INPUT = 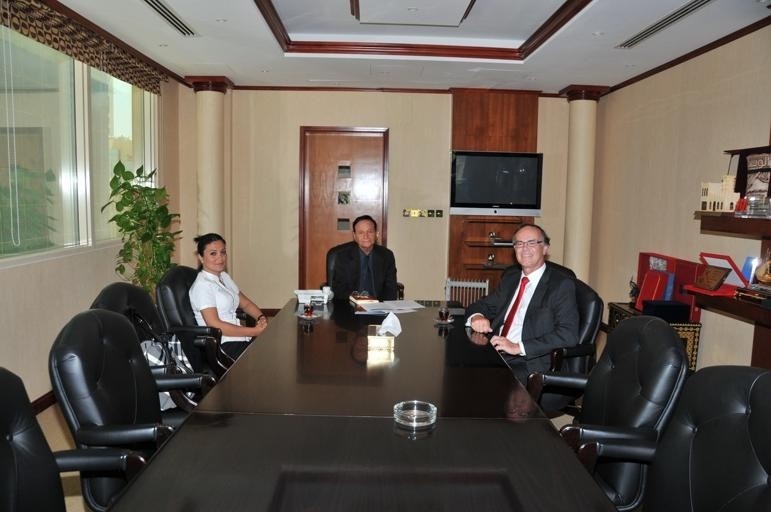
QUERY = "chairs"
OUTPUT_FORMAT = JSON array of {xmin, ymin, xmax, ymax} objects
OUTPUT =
[
  {"xmin": 324, "ymin": 239, "xmax": 405, "ymax": 301},
  {"xmin": 49, "ymin": 308, "xmax": 191, "ymax": 512},
  {"xmin": 87, "ymin": 280, "xmax": 219, "ymax": 421},
  {"xmin": 526, "ymin": 276, "xmax": 603, "ymax": 414},
  {"xmin": 0, "ymin": 367, "xmax": 146, "ymax": 512},
  {"xmin": 577, "ymin": 364, "xmax": 770, "ymax": 511},
  {"xmin": 529, "ymin": 315, "xmax": 689, "ymax": 511},
  {"xmin": 154, "ymin": 265, "xmax": 252, "ymax": 369}
]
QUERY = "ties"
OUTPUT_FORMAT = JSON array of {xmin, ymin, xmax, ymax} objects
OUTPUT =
[
  {"xmin": 363, "ymin": 255, "xmax": 374, "ymax": 296},
  {"xmin": 501, "ymin": 277, "xmax": 530, "ymax": 338}
]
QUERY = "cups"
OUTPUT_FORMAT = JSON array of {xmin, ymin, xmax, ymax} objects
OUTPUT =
[
  {"xmin": 304, "ymin": 303, "xmax": 314, "ymax": 316},
  {"xmin": 438, "ymin": 307, "xmax": 450, "ymax": 322}
]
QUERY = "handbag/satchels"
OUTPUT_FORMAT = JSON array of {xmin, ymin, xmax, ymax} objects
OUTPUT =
[{"xmin": 125, "ymin": 306, "xmax": 196, "ymax": 411}]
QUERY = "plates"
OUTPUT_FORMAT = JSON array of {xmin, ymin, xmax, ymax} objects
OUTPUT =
[
  {"xmin": 298, "ymin": 314, "xmax": 318, "ymax": 320},
  {"xmin": 434, "ymin": 319, "xmax": 454, "ymax": 324}
]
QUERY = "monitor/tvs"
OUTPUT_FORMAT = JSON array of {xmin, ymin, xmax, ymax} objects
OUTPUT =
[{"xmin": 448, "ymin": 150, "xmax": 544, "ymax": 217}]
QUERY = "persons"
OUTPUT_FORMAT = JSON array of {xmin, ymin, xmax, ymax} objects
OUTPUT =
[
  {"xmin": 464, "ymin": 224, "xmax": 579, "ymax": 387},
  {"xmin": 331, "ymin": 215, "xmax": 397, "ymax": 302},
  {"xmin": 187, "ymin": 234, "xmax": 268, "ymax": 361}
]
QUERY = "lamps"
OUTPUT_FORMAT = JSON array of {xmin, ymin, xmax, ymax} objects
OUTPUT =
[{"xmin": 359, "ymin": 0, "xmax": 472, "ymax": 27}]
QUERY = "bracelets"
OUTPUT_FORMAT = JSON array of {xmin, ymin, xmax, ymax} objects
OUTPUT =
[{"xmin": 258, "ymin": 314, "xmax": 267, "ymax": 320}]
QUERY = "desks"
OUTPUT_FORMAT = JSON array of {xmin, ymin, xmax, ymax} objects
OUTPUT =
[
  {"xmin": 97, "ymin": 416, "xmax": 617, "ymax": 512},
  {"xmin": 190, "ymin": 298, "xmax": 546, "ymax": 419}
]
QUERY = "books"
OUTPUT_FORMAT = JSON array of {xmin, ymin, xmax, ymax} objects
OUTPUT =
[{"xmin": 348, "ymin": 294, "xmax": 379, "ymax": 304}]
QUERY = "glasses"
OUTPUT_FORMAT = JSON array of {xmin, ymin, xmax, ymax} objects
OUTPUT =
[{"xmin": 513, "ymin": 239, "xmax": 546, "ymax": 247}]
